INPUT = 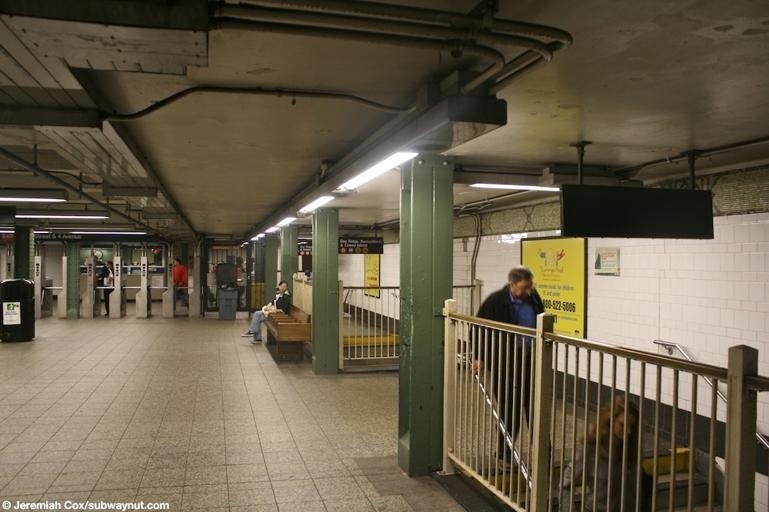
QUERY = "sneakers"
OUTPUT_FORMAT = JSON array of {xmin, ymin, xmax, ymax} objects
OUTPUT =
[
  {"xmin": 497, "ymin": 458, "xmax": 514, "ymax": 472},
  {"xmin": 241, "ymin": 329, "xmax": 263, "ymax": 344}
]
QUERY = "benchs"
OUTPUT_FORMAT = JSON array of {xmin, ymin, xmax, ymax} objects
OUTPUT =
[{"xmin": 262, "ymin": 304, "xmax": 313, "ymax": 362}]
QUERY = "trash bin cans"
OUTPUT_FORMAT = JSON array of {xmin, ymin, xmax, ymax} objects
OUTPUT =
[
  {"xmin": 216, "ymin": 263, "xmax": 239, "ymax": 321},
  {"xmin": 1, "ymin": 279, "xmax": 35, "ymax": 342}
]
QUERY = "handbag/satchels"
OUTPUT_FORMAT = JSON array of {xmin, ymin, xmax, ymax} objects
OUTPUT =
[{"xmin": 102, "ymin": 270, "xmax": 114, "ymax": 288}]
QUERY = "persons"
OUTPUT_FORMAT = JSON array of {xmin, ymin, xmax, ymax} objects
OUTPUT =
[
  {"xmin": 241, "ymin": 280, "xmax": 291, "ymax": 344},
  {"xmin": 559, "ymin": 395, "xmax": 639, "ymax": 512},
  {"xmin": 235, "ymin": 256, "xmax": 247, "ymax": 305},
  {"xmin": 469, "ymin": 265, "xmax": 551, "ymax": 476},
  {"xmin": 173, "ymin": 258, "xmax": 189, "ymax": 305},
  {"xmin": 103, "ymin": 261, "xmax": 114, "ymax": 318}
]
